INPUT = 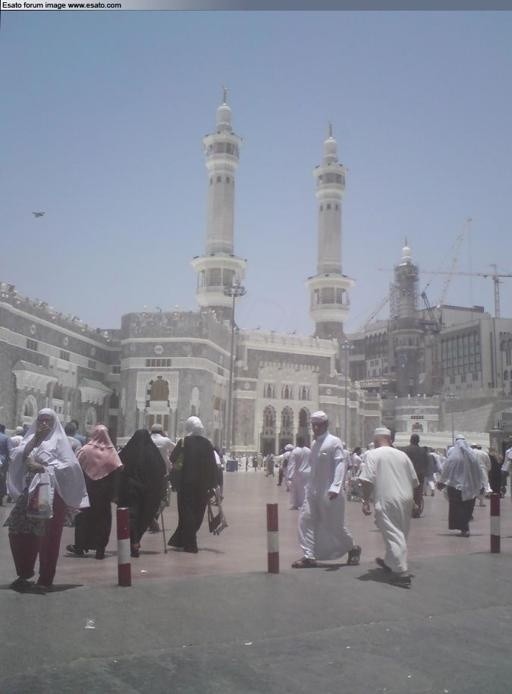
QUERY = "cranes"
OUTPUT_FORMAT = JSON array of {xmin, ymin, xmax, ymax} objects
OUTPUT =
[{"xmin": 360, "ymin": 216, "xmax": 512, "ymax": 330}]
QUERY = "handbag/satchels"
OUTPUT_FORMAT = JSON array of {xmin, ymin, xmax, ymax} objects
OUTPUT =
[
  {"xmin": 169, "ymin": 447, "xmax": 184, "ymax": 492},
  {"xmin": 26, "ymin": 466, "xmax": 55, "ymax": 519}
]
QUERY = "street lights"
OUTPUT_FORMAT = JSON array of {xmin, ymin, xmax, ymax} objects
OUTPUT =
[
  {"xmin": 446, "ymin": 390, "xmax": 457, "ymax": 447},
  {"xmin": 341, "ymin": 338, "xmax": 355, "ymax": 450},
  {"xmin": 223, "ymin": 278, "xmax": 246, "ymax": 456}
]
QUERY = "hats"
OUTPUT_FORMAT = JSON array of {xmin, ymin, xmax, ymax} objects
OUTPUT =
[
  {"xmin": 310, "ymin": 410, "xmax": 328, "ymax": 423},
  {"xmin": 374, "ymin": 428, "xmax": 391, "ymax": 436}
]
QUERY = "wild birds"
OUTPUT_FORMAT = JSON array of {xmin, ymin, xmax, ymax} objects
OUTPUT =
[{"xmin": 32, "ymin": 212, "xmax": 45, "ymax": 217}]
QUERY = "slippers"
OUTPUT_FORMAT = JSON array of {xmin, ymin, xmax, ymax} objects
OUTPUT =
[{"xmin": 67, "ymin": 544, "xmax": 84, "ymax": 555}]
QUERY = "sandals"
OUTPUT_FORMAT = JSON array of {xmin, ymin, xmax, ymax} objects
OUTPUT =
[
  {"xmin": 292, "ymin": 557, "xmax": 318, "ymax": 568},
  {"xmin": 347, "ymin": 546, "xmax": 361, "ymax": 565}
]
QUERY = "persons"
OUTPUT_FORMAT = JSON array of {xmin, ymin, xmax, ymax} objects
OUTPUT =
[{"xmin": 1, "ymin": 408, "xmax": 512, "ymax": 593}]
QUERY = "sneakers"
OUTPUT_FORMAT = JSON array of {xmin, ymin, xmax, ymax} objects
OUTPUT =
[
  {"xmin": 376, "ymin": 558, "xmax": 411, "ymax": 586},
  {"xmin": 11, "ymin": 576, "xmax": 50, "ymax": 594}
]
